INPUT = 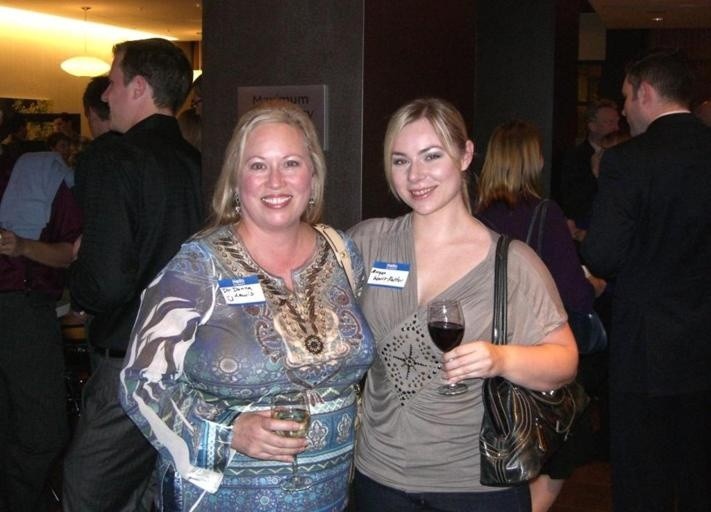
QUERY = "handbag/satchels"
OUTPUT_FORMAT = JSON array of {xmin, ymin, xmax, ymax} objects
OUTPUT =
[
  {"xmin": 523, "ymin": 197, "xmax": 608, "ymax": 353},
  {"xmin": 475, "ymin": 229, "xmax": 590, "ymax": 490}
]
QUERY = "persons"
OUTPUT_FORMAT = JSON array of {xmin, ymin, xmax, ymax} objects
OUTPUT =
[
  {"xmin": 117, "ymin": 100, "xmax": 377, "ymax": 512},
  {"xmin": 343, "ymin": 97, "xmax": 582, "ymax": 512},
  {"xmin": 474, "ymin": 118, "xmax": 607, "ymax": 512},
  {"xmin": 60, "ymin": 35, "xmax": 201, "ymax": 511},
  {"xmin": 551, "ymin": 96, "xmax": 710, "ymax": 242},
  {"xmin": 579, "ymin": 45, "xmax": 711, "ymax": 512}
]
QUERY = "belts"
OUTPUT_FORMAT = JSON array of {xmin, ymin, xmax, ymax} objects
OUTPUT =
[{"xmin": 92, "ymin": 346, "xmax": 126, "ymax": 360}]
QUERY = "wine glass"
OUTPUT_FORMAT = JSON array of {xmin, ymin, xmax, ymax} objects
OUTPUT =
[
  {"xmin": 425, "ymin": 297, "xmax": 469, "ymax": 398},
  {"xmin": 268, "ymin": 389, "xmax": 318, "ymax": 495}
]
